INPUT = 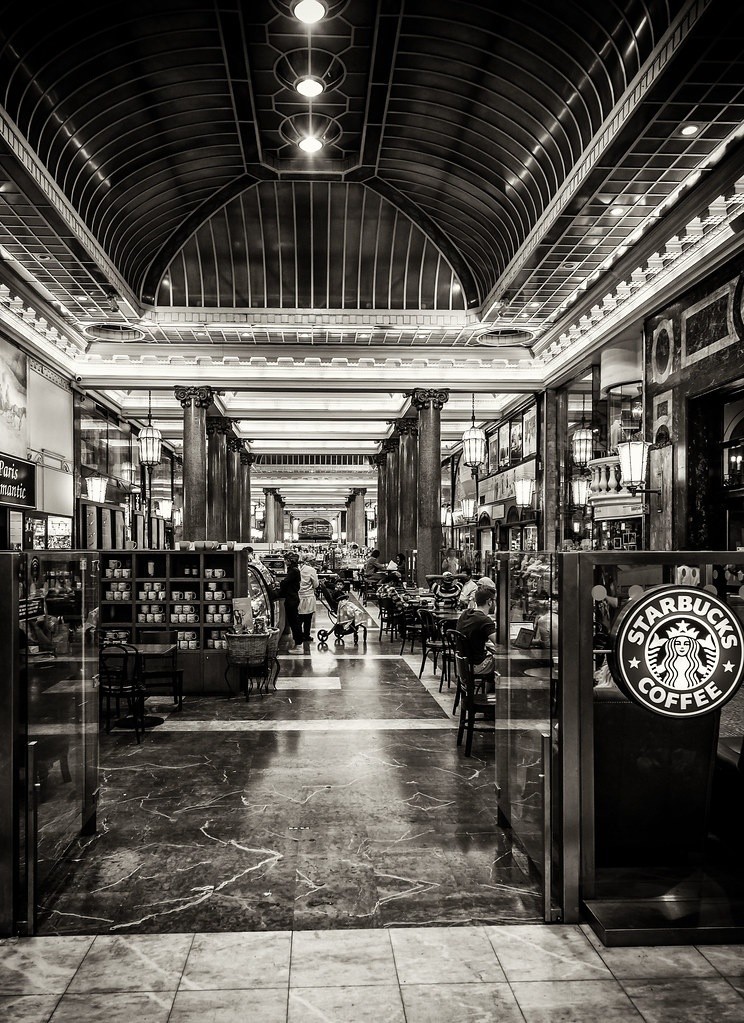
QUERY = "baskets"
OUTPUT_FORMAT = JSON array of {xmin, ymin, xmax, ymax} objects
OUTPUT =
[
  {"xmin": 266, "ymin": 627, "xmax": 280, "ymax": 659},
  {"xmin": 227, "ymin": 632, "xmax": 268, "ymax": 665}
]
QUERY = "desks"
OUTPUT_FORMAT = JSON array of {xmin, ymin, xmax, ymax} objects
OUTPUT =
[
  {"xmin": 436, "ymin": 609, "xmax": 463, "ymax": 624},
  {"xmin": 101, "ymin": 642, "xmax": 177, "ymax": 728},
  {"xmin": 484, "ymin": 643, "xmax": 550, "ymax": 678}
]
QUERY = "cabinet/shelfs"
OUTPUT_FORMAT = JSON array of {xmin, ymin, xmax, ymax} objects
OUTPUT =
[{"xmin": 70, "ymin": 548, "xmax": 253, "ymax": 691}]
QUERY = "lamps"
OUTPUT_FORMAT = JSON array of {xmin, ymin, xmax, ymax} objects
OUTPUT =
[
  {"xmin": 363, "ymin": 394, "xmax": 642, "ymax": 549},
  {"xmin": 86, "ymin": 386, "xmax": 300, "ymax": 541}
]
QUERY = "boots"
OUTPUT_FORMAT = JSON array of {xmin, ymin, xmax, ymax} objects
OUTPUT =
[{"xmin": 288, "ymin": 643, "xmax": 304, "ymax": 655}]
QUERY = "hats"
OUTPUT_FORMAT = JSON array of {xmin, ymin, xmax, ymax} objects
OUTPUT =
[
  {"xmin": 303, "ymin": 553, "xmax": 316, "ymax": 562},
  {"xmin": 476, "ymin": 576, "xmax": 496, "ymax": 588},
  {"xmin": 475, "ymin": 584, "xmax": 495, "ymax": 600}
]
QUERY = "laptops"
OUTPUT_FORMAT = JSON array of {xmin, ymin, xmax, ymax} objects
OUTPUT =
[{"xmin": 509, "ymin": 621, "xmax": 534, "ymax": 650}]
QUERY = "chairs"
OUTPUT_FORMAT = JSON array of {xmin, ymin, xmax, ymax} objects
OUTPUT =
[
  {"xmin": 96, "ymin": 629, "xmax": 183, "ymax": 740},
  {"xmin": 356, "ymin": 566, "xmax": 496, "ymax": 757}
]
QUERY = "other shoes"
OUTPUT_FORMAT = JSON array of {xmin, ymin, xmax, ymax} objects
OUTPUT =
[{"xmin": 304, "ymin": 635, "xmax": 313, "ymax": 641}]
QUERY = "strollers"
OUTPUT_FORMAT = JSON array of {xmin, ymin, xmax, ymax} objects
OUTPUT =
[{"xmin": 314, "ymin": 578, "xmax": 368, "ymax": 646}]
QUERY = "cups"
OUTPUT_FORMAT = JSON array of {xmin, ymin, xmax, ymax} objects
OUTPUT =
[
  {"xmin": 204, "ymin": 583, "xmax": 233, "ymax": 600},
  {"xmin": 192, "ymin": 569, "xmax": 198, "ymax": 575},
  {"xmin": 105, "ymin": 560, "xmax": 131, "ymax": 578},
  {"xmin": 125, "ymin": 540, "xmax": 137, "ymax": 550},
  {"xmin": 205, "ymin": 568, "xmax": 214, "ymax": 578},
  {"xmin": 137, "ymin": 605, "xmax": 165, "ymax": 622},
  {"xmin": 139, "ymin": 582, "xmax": 166, "ymax": 601},
  {"xmin": 227, "ymin": 541, "xmax": 236, "ymax": 551},
  {"xmin": 207, "ymin": 630, "xmax": 229, "ymax": 649},
  {"xmin": 185, "ymin": 592, "xmax": 196, "ymax": 600},
  {"xmin": 170, "ymin": 605, "xmax": 199, "ymax": 623},
  {"xmin": 184, "ymin": 568, "xmax": 190, "ymax": 574},
  {"xmin": 215, "ymin": 569, "xmax": 226, "ymax": 578},
  {"xmin": 102, "ymin": 629, "xmax": 130, "ymax": 646},
  {"xmin": 179, "ymin": 540, "xmax": 193, "ymax": 550},
  {"xmin": 176, "ymin": 631, "xmax": 200, "ymax": 650},
  {"xmin": 193, "ymin": 541, "xmax": 219, "ymax": 551},
  {"xmin": 206, "ymin": 605, "xmax": 231, "ymax": 622},
  {"xmin": 172, "ymin": 591, "xmax": 184, "ymax": 600},
  {"xmin": 582, "ymin": 538, "xmax": 592, "ymax": 551},
  {"xmin": 106, "ymin": 583, "xmax": 132, "ymax": 600}
]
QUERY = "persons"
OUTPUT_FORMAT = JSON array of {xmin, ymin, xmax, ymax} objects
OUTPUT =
[
  {"xmin": 455, "ymin": 584, "xmax": 496, "ymax": 674},
  {"xmin": 531, "ymin": 603, "xmax": 559, "ymax": 648},
  {"xmin": 442, "ymin": 548, "xmax": 461, "ymax": 574},
  {"xmin": 246, "ymin": 547, "xmax": 259, "ymax": 559},
  {"xmin": 425, "ymin": 567, "xmax": 477, "ymax": 609},
  {"xmin": 363, "ymin": 550, "xmax": 387, "ymax": 585},
  {"xmin": 273, "ymin": 551, "xmax": 303, "ymax": 645},
  {"xmin": 395, "ymin": 553, "xmax": 405, "ymax": 575},
  {"xmin": 332, "ymin": 579, "xmax": 345, "ymax": 600},
  {"xmin": 299, "ymin": 553, "xmax": 319, "ymax": 642}
]
